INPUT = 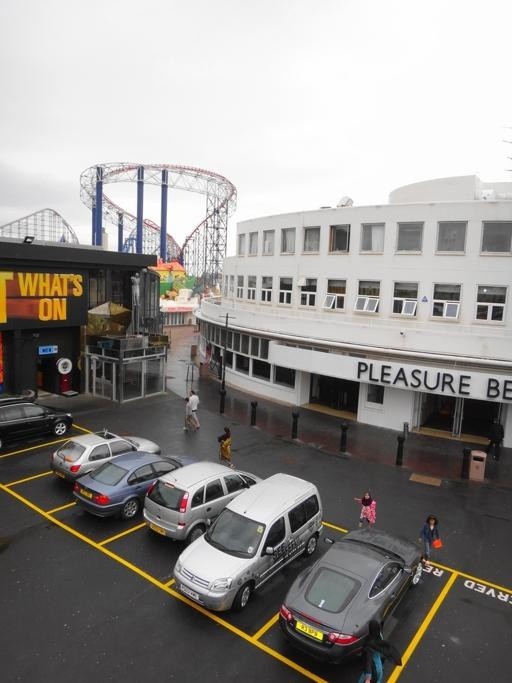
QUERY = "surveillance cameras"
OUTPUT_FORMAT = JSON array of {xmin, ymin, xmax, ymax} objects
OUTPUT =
[{"xmin": 399, "ymin": 331, "xmax": 405, "ymax": 336}]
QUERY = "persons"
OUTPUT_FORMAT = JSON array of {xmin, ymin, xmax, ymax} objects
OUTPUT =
[
  {"xmin": 353, "ymin": 490, "xmax": 376, "ymax": 528},
  {"xmin": 218, "ymin": 427, "xmax": 234, "ymax": 468},
  {"xmin": 485, "ymin": 417, "xmax": 504, "ymax": 461},
  {"xmin": 189, "ymin": 391, "xmax": 200, "ymax": 430},
  {"xmin": 130, "ymin": 273, "xmax": 141, "ymax": 306},
  {"xmin": 357, "ymin": 620, "xmax": 402, "ymax": 683},
  {"xmin": 183, "ymin": 397, "xmax": 196, "ymax": 433},
  {"xmin": 418, "ymin": 514, "xmax": 440, "ymax": 561}
]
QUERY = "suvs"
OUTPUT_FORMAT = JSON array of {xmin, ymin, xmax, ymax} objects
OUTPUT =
[
  {"xmin": 52, "ymin": 428, "xmax": 161, "ymax": 485},
  {"xmin": 0, "ymin": 397, "xmax": 74, "ymax": 446},
  {"xmin": 143, "ymin": 460, "xmax": 263, "ymax": 544},
  {"xmin": 171, "ymin": 472, "xmax": 324, "ymax": 613},
  {"xmin": 280, "ymin": 526, "xmax": 428, "ymax": 664}
]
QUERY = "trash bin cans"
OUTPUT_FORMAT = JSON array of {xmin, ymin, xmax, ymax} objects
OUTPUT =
[
  {"xmin": 469, "ymin": 450, "xmax": 488, "ymax": 481},
  {"xmin": 200, "ymin": 360, "xmax": 209, "ymax": 376}
]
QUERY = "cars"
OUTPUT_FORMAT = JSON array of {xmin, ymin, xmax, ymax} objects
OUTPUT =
[{"xmin": 72, "ymin": 449, "xmax": 200, "ymax": 520}]
